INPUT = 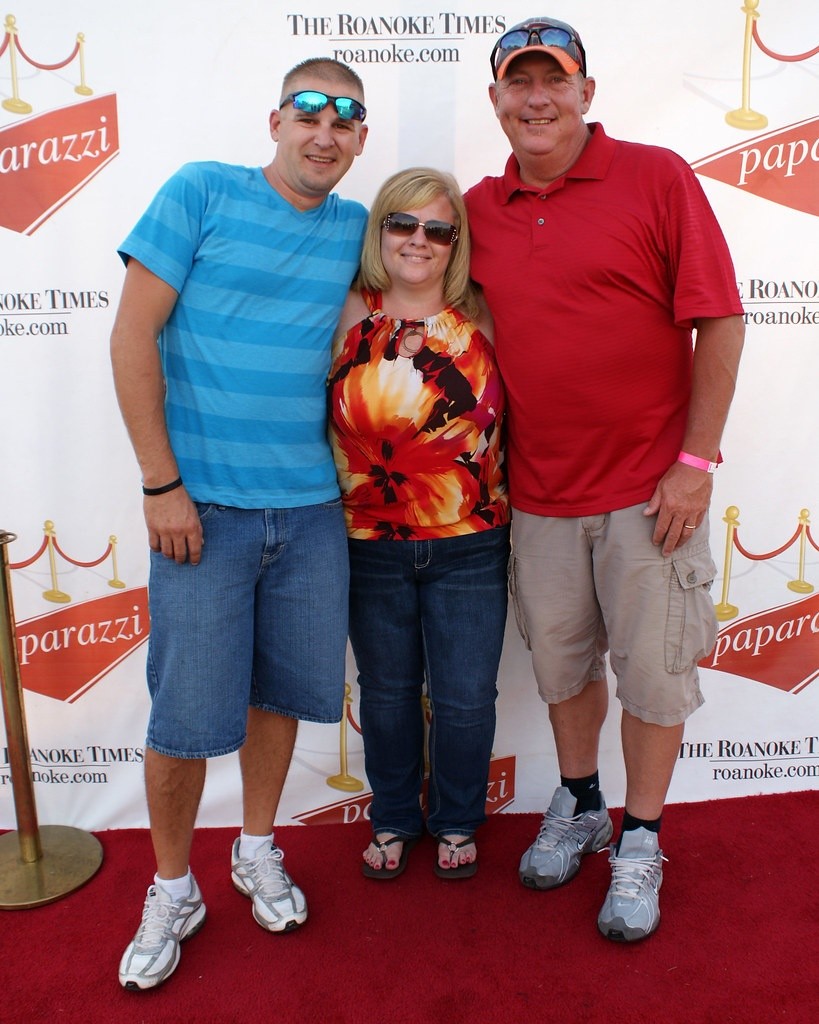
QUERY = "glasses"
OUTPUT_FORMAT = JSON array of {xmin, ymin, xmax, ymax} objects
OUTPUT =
[
  {"xmin": 490, "ymin": 26, "xmax": 587, "ymax": 82},
  {"xmin": 279, "ymin": 89, "xmax": 367, "ymax": 123},
  {"xmin": 381, "ymin": 212, "xmax": 459, "ymax": 246}
]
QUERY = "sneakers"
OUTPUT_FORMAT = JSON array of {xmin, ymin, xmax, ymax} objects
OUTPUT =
[
  {"xmin": 598, "ymin": 826, "xmax": 669, "ymax": 943},
  {"xmin": 231, "ymin": 837, "xmax": 308, "ymax": 935},
  {"xmin": 118, "ymin": 873, "xmax": 206, "ymax": 991},
  {"xmin": 518, "ymin": 786, "xmax": 613, "ymax": 889}
]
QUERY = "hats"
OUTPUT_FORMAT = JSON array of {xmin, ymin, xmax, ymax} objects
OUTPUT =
[{"xmin": 495, "ymin": 16, "xmax": 585, "ymax": 81}]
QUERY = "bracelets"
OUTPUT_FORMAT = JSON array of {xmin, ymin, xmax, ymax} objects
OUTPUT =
[
  {"xmin": 677, "ymin": 451, "xmax": 721, "ymax": 475},
  {"xmin": 140, "ymin": 475, "xmax": 184, "ymax": 495}
]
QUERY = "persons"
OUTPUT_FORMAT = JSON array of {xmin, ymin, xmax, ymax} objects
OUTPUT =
[
  {"xmin": 323, "ymin": 165, "xmax": 515, "ymax": 881},
  {"xmin": 104, "ymin": 59, "xmax": 371, "ymax": 990},
  {"xmin": 457, "ymin": 13, "xmax": 745, "ymax": 946}
]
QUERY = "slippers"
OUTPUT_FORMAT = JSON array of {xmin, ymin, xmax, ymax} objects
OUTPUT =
[
  {"xmin": 361, "ymin": 827, "xmax": 409, "ymax": 878},
  {"xmin": 434, "ymin": 833, "xmax": 478, "ymax": 878}
]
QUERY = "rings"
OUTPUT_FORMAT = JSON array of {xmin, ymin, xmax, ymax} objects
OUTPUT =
[{"xmin": 684, "ymin": 524, "xmax": 697, "ymax": 530}]
